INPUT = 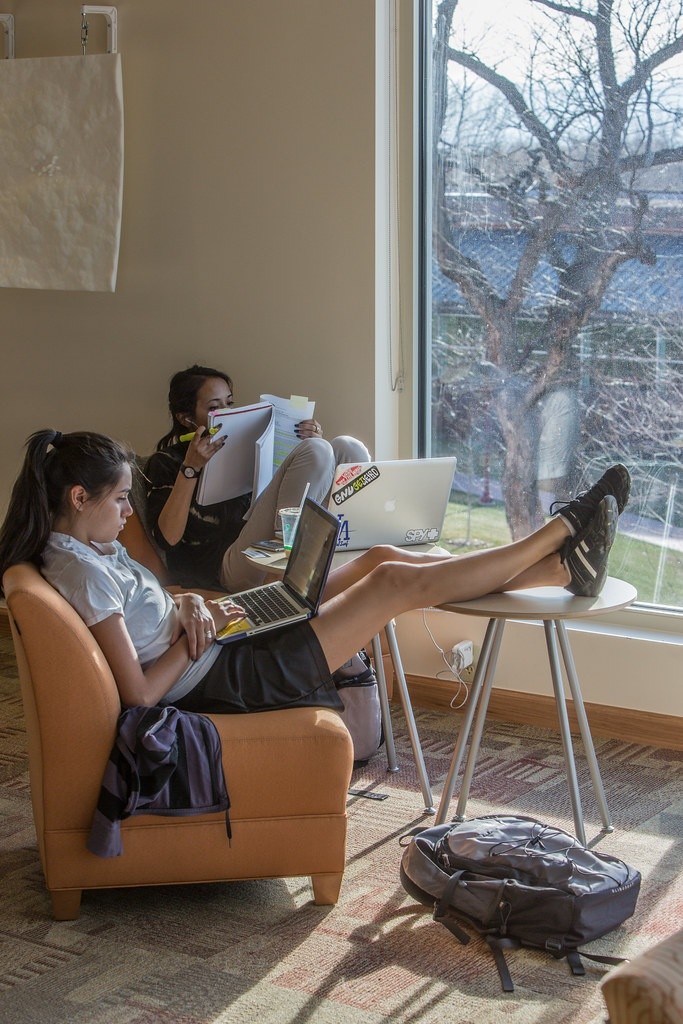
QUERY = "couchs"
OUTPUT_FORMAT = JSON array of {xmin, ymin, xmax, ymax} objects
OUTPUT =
[
  {"xmin": 2, "ymin": 560, "xmax": 354, "ymax": 921},
  {"xmin": 118, "ymin": 454, "xmax": 394, "ymax": 700}
]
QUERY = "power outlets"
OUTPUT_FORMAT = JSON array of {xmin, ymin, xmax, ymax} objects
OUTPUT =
[{"xmin": 459, "ymin": 645, "xmax": 479, "ymax": 684}]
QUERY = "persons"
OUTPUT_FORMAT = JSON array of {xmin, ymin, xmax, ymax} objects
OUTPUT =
[
  {"xmin": 1, "ymin": 429, "xmax": 632, "ymax": 714},
  {"xmin": 142, "ymin": 365, "xmax": 371, "ymax": 594}
]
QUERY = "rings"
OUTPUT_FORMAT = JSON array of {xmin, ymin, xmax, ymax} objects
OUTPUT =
[{"xmin": 205, "ymin": 630, "xmax": 213, "ymax": 638}]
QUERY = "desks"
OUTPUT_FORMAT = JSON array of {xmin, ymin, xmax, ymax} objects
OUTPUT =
[
  {"xmin": 434, "ymin": 574, "xmax": 638, "ymax": 849},
  {"xmin": 247, "ymin": 544, "xmax": 451, "ymax": 814}
]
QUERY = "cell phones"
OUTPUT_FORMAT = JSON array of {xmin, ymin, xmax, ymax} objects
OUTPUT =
[{"xmin": 250, "ymin": 541, "xmax": 284, "ymax": 553}]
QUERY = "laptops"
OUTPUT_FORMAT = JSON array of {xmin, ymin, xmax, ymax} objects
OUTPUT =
[
  {"xmin": 274, "ymin": 457, "xmax": 458, "ymax": 552},
  {"xmin": 205, "ymin": 498, "xmax": 343, "ymax": 646}
]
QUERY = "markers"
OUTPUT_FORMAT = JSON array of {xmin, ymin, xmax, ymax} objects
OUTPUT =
[{"xmin": 180, "ymin": 428, "xmax": 219, "ymax": 442}]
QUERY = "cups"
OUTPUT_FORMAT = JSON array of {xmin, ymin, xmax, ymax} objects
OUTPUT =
[{"xmin": 278, "ymin": 507, "xmax": 300, "ymax": 558}]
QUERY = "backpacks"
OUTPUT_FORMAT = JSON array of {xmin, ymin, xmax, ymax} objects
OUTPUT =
[
  {"xmin": 332, "ymin": 649, "xmax": 384, "ymax": 771},
  {"xmin": 397, "ymin": 814, "xmax": 641, "ymax": 992}
]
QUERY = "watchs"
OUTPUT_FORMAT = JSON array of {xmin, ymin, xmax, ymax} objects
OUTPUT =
[{"xmin": 180, "ymin": 464, "xmax": 203, "ymax": 479}]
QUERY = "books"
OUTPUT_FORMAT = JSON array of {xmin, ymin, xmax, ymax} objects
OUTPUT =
[{"xmin": 196, "ymin": 393, "xmax": 315, "ymax": 506}]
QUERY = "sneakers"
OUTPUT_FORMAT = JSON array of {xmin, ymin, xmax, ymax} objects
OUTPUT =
[
  {"xmin": 560, "ymin": 495, "xmax": 619, "ymax": 598},
  {"xmin": 549, "ymin": 463, "xmax": 631, "ymax": 534}
]
